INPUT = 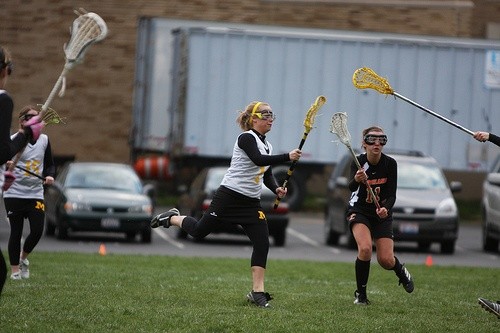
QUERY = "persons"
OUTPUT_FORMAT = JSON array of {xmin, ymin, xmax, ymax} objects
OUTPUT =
[
  {"xmin": 346, "ymin": 127, "xmax": 414, "ymax": 306},
  {"xmin": 152, "ymin": 102, "xmax": 301, "ymax": 307},
  {"xmin": 0, "ymin": 46, "xmax": 44, "ymax": 293},
  {"xmin": 0, "ymin": 104, "xmax": 56, "ymax": 280},
  {"xmin": 473, "ymin": 131, "xmax": 500, "ymax": 319}
]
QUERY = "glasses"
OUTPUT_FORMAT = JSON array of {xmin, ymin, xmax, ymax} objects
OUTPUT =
[
  {"xmin": 364, "ymin": 134, "xmax": 388, "ymax": 145},
  {"xmin": 0, "ymin": 61, "xmax": 11, "ymax": 76},
  {"xmin": 22, "ymin": 114, "xmax": 36, "ymax": 121},
  {"xmin": 250, "ymin": 113, "xmax": 277, "ymax": 120}
]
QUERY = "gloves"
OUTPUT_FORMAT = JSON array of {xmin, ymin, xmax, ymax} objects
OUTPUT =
[{"xmin": 20, "ymin": 117, "xmax": 46, "ymax": 145}]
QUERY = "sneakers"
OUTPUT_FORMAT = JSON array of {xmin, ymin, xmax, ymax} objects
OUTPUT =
[
  {"xmin": 246, "ymin": 290, "xmax": 274, "ymax": 308},
  {"xmin": 354, "ymin": 289, "xmax": 370, "ymax": 304},
  {"xmin": 20, "ymin": 258, "xmax": 29, "ymax": 278},
  {"xmin": 395, "ymin": 264, "xmax": 414, "ymax": 294},
  {"xmin": 478, "ymin": 297, "xmax": 500, "ymax": 319},
  {"xmin": 11, "ymin": 269, "xmax": 21, "ymax": 279},
  {"xmin": 151, "ymin": 207, "xmax": 180, "ymax": 228}
]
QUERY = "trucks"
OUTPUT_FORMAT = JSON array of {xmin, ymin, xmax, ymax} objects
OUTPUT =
[{"xmin": 127, "ymin": 15, "xmax": 500, "ymax": 181}]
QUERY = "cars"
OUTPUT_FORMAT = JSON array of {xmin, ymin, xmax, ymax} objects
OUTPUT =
[
  {"xmin": 188, "ymin": 167, "xmax": 290, "ymax": 246},
  {"xmin": 481, "ymin": 153, "xmax": 500, "ymax": 250},
  {"xmin": 45, "ymin": 161, "xmax": 154, "ymax": 243}
]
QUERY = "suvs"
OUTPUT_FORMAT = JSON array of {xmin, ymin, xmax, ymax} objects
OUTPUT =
[{"xmin": 324, "ymin": 149, "xmax": 463, "ymax": 255}]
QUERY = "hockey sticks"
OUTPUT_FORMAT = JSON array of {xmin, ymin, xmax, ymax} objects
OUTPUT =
[
  {"xmin": 352, "ymin": 66, "xmax": 486, "ymax": 144},
  {"xmin": 329, "ymin": 112, "xmax": 380, "ymax": 211},
  {"xmin": 9, "ymin": 104, "xmax": 64, "ymax": 172},
  {"xmin": 15, "ymin": 165, "xmax": 47, "ymax": 181},
  {"xmin": 272, "ymin": 94, "xmax": 327, "ymax": 210},
  {"xmin": 38, "ymin": 12, "xmax": 109, "ymax": 119}
]
